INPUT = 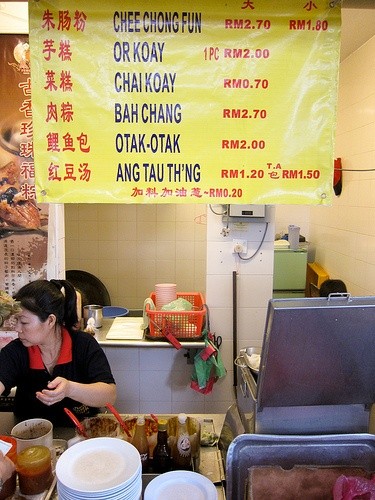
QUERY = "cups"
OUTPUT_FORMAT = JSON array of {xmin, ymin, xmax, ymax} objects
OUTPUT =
[
  {"xmin": 83, "ymin": 304, "xmax": 103, "ymax": 328},
  {"xmin": 15, "ymin": 445, "xmax": 52, "ymax": 495},
  {"xmin": 11, "ymin": 418, "xmax": 54, "ymax": 461},
  {"xmin": 117, "ymin": 415, "xmax": 159, "ymax": 458},
  {"xmin": 76, "ymin": 416, "xmax": 119, "ymax": 438},
  {"xmin": 0, "ymin": 434, "xmax": 18, "ymax": 464},
  {"xmin": 162, "ymin": 416, "xmax": 202, "ymax": 471},
  {"xmin": 155, "ymin": 283, "xmax": 176, "ymax": 312}
]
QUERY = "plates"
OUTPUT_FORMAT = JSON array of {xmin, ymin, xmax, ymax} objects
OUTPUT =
[{"xmin": 55, "ymin": 437, "xmax": 218, "ymax": 500}]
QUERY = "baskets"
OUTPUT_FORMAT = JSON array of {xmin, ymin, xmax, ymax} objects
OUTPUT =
[{"xmin": 146, "ymin": 292, "xmax": 206, "ymax": 338}]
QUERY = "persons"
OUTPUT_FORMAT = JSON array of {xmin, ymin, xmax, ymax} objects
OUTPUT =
[{"xmin": 0, "ymin": 279, "xmax": 118, "ymax": 428}]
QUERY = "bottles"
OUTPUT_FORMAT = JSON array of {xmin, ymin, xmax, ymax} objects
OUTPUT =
[
  {"xmin": 153, "ymin": 419, "xmax": 172, "ymax": 474},
  {"xmin": 173, "ymin": 413, "xmax": 191, "ymax": 471},
  {"xmin": 131, "ymin": 416, "xmax": 150, "ymax": 473}
]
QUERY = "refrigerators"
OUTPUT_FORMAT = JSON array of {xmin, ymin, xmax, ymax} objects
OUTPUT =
[{"xmin": 272, "ymin": 247, "xmax": 307, "ymax": 299}]
